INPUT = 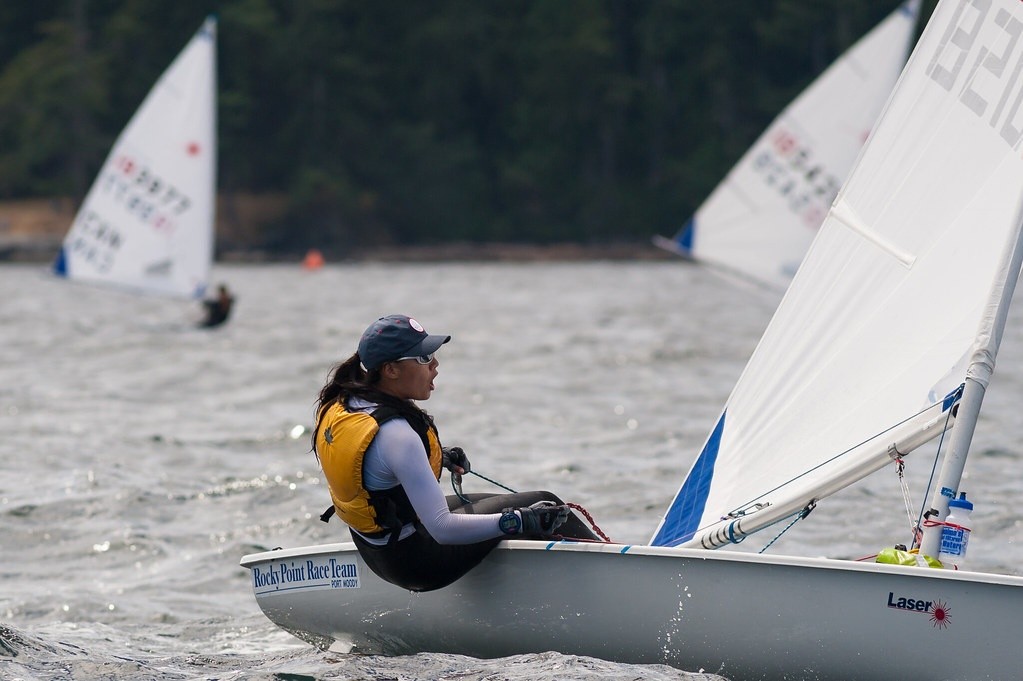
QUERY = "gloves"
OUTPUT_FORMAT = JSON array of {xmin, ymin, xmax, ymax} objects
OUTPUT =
[
  {"xmin": 516, "ymin": 501, "xmax": 570, "ymax": 536},
  {"xmin": 441, "ymin": 446, "xmax": 471, "ymax": 475}
]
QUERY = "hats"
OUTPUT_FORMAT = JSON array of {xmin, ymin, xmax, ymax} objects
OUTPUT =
[{"xmin": 358, "ymin": 314, "xmax": 451, "ymax": 370}]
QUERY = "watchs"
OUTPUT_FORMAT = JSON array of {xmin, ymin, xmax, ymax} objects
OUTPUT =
[{"xmin": 499, "ymin": 507, "xmax": 521, "ymax": 536}]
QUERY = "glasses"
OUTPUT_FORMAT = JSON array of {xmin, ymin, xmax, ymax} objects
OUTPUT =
[{"xmin": 396, "ymin": 353, "xmax": 435, "ymax": 365}]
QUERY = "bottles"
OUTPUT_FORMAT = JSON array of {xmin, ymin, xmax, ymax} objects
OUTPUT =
[{"xmin": 937, "ymin": 492, "xmax": 974, "ymax": 566}]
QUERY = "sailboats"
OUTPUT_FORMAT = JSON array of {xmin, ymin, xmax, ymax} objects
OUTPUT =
[
  {"xmin": 39, "ymin": 13, "xmax": 216, "ymax": 336},
  {"xmin": 236, "ymin": 2, "xmax": 1023, "ymax": 680}
]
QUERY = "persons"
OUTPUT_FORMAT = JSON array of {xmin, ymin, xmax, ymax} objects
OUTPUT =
[
  {"xmin": 307, "ymin": 314, "xmax": 602, "ymax": 593},
  {"xmin": 194, "ymin": 285, "xmax": 233, "ymax": 329}
]
